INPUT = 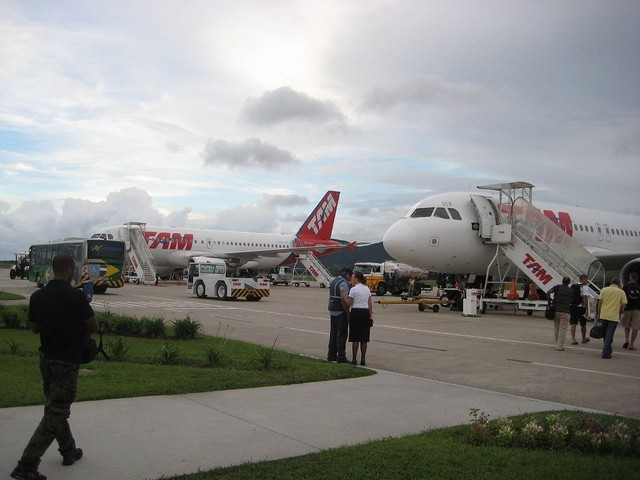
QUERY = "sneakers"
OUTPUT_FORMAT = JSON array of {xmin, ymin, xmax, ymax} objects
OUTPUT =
[
  {"xmin": 63, "ymin": 448, "xmax": 83, "ymax": 465},
  {"xmin": 11, "ymin": 467, "xmax": 47, "ymax": 480},
  {"xmin": 629, "ymin": 344, "xmax": 637, "ymax": 349},
  {"xmin": 623, "ymin": 343, "xmax": 629, "ymax": 348}
]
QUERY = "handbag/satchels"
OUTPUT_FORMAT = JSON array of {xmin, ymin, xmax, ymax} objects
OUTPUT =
[
  {"xmin": 590, "ymin": 325, "xmax": 604, "ymax": 338},
  {"xmin": 80, "ymin": 338, "xmax": 99, "ymax": 363},
  {"xmin": 545, "ymin": 303, "xmax": 555, "ymax": 320}
]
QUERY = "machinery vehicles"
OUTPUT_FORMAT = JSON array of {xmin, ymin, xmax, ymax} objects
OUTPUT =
[{"xmin": 7, "ymin": 251, "xmax": 30, "ymax": 280}]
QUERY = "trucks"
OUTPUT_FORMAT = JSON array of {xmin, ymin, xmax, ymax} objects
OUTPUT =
[
  {"xmin": 187, "ymin": 256, "xmax": 270, "ymax": 300},
  {"xmin": 350, "ymin": 260, "xmax": 428, "ymax": 295}
]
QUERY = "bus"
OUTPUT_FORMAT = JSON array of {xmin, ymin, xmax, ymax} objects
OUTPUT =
[{"xmin": 29, "ymin": 238, "xmax": 125, "ymax": 295}]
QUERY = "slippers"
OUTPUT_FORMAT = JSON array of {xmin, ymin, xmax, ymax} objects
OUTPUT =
[
  {"xmin": 572, "ymin": 342, "xmax": 578, "ymax": 345},
  {"xmin": 582, "ymin": 339, "xmax": 590, "ymax": 343}
]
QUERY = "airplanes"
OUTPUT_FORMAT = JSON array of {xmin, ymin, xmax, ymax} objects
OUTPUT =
[
  {"xmin": 382, "ymin": 180, "xmax": 640, "ymax": 317},
  {"xmin": 90, "ymin": 190, "xmax": 356, "ymax": 288}
]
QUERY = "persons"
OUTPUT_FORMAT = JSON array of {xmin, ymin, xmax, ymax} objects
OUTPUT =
[
  {"xmin": 340, "ymin": 272, "xmax": 373, "ymax": 365},
  {"xmin": 401, "ymin": 288, "xmax": 409, "ymax": 302},
  {"xmin": 11, "ymin": 253, "xmax": 100, "ymax": 480},
  {"xmin": 327, "ymin": 266, "xmax": 352, "ymax": 361},
  {"xmin": 546, "ymin": 276, "xmax": 575, "ymax": 351},
  {"xmin": 621, "ymin": 273, "xmax": 640, "ymax": 350},
  {"xmin": 595, "ymin": 278, "xmax": 628, "ymax": 358},
  {"xmin": 521, "ymin": 278, "xmax": 530, "ymax": 300},
  {"xmin": 570, "ymin": 274, "xmax": 590, "ymax": 345},
  {"xmin": 407, "ymin": 276, "xmax": 416, "ymax": 296}
]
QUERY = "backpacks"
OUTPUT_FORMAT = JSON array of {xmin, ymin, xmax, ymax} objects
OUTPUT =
[{"xmin": 571, "ymin": 283, "xmax": 586, "ymax": 305}]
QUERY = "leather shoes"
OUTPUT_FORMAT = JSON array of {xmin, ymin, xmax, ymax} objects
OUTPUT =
[
  {"xmin": 337, "ymin": 358, "xmax": 351, "ymax": 363},
  {"xmin": 328, "ymin": 357, "xmax": 338, "ymax": 361}
]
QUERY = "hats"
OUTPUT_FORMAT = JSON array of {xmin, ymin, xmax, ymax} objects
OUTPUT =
[{"xmin": 342, "ymin": 267, "xmax": 354, "ymax": 274}]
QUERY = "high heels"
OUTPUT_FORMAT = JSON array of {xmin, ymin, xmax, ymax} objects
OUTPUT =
[{"xmin": 352, "ymin": 360, "xmax": 357, "ymax": 366}]
